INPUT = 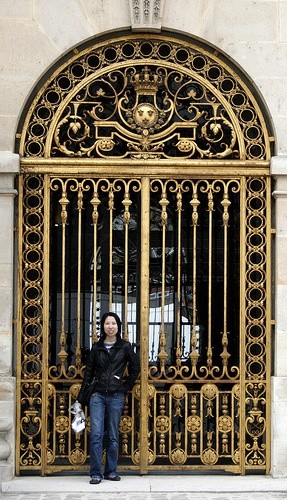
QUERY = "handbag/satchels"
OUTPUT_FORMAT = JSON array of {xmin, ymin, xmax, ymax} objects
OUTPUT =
[{"xmin": 70, "ymin": 401, "xmax": 86, "ymax": 436}]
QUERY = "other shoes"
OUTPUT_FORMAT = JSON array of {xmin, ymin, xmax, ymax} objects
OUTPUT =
[
  {"xmin": 103, "ymin": 472, "xmax": 120, "ymax": 481},
  {"xmin": 88, "ymin": 475, "xmax": 101, "ymax": 484}
]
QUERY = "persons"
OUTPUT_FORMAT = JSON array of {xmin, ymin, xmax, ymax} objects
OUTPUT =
[{"xmin": 74, "ymin": 312, "xmax": 141, "ymax": 485}]
